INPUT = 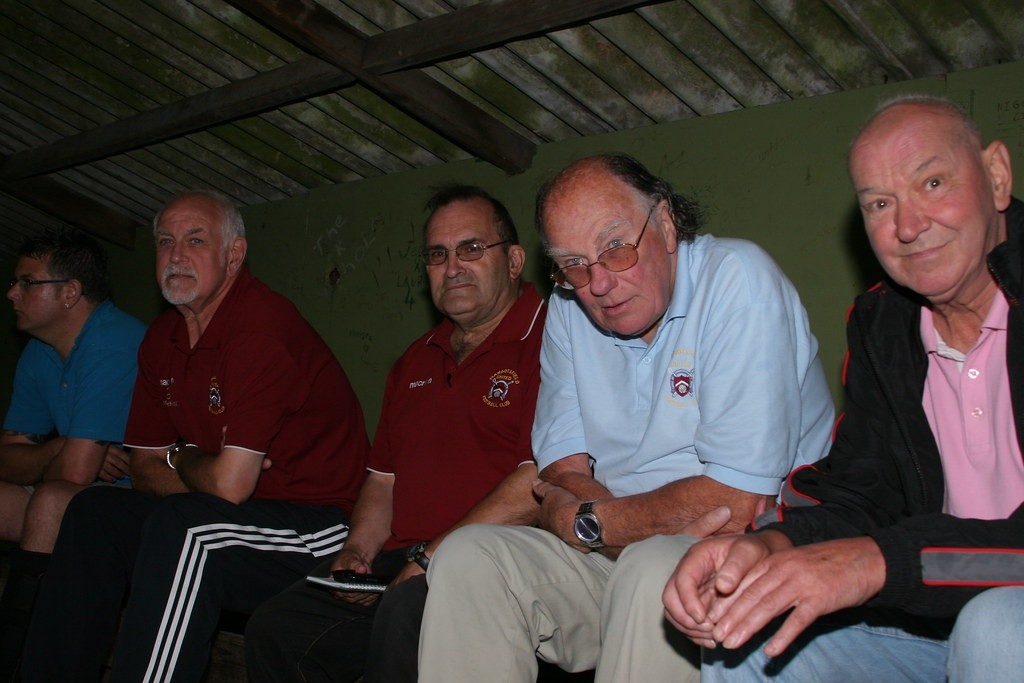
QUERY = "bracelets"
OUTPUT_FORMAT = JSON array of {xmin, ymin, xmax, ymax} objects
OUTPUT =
[{"xmin": 167, "ymin": 443, "xmax": 197, "ymax": 470}]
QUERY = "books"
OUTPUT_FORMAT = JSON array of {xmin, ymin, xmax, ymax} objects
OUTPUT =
[{"xmin": 307, "ymin": 574, "xmax": 387, "ymax": 593}]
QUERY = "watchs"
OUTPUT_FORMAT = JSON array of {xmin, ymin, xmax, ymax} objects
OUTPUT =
[
  {"xmin": 408, "ymin": 541, "xmax": 430, "ymax": 571},
  {"xmin": 574, "ymin": 502, "xmax": 606, "ymax": 547}
]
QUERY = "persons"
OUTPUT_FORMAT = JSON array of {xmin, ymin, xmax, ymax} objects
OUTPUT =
[
  {"xmin": 0, "ymin": 191, "xmax": 374, "ymax": 683},
  {"xmin": 661, "ymin": 94, "xmax": 1024, "ymax": 683},
  {"xmin": 0, "ymin": 241, "xmax": 149, "ymax": 683},
  {"xmin": 417, "ymin": 151, "xmax": 833, "ymax": 683},
  {"xmin": 242, "ymin": 185, "xmax": 548, "ymax": 683}
]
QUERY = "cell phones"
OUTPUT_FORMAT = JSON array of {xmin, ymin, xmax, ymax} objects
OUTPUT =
[{"xmin": 332, "ymin": 569, "xmax": 388, "ymax": 585}]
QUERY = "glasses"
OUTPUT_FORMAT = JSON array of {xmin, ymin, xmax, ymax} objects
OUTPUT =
[
  {"xmin": 551, "ymin": 205, "xmax": 656, "ymax": 290},
  {"xmin": 419, "ymin": 239, "xmax": 511, "ymax": 266},
  {"xmin": 9, "ymin": 278, "xmax": 69, "ymax": 291}
]
